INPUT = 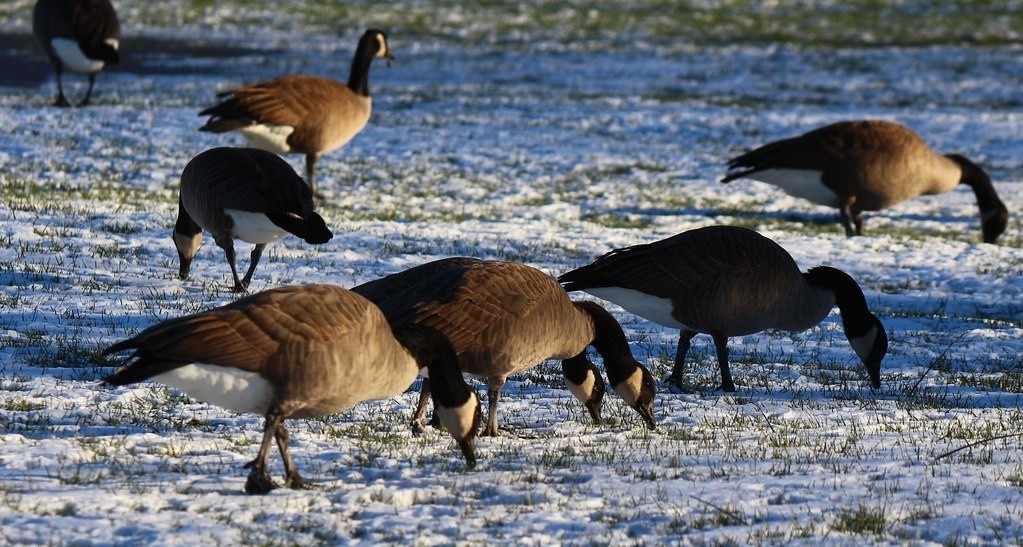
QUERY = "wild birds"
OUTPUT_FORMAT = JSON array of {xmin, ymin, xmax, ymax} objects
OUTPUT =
[
  {"xmin": 720, "ymin": 120, "xmax": 1011, "ymax": 243},
  {"xmin": 177, "ymin": 146, "xmax": 333, "ymax": 294},
  {"xmin": 94, "ymin": 224, "xmax": 889, "ymax": 494},
  {"xmin": 197, "ymin": 28, "xmax": 397, "ymax": 200},
  {"xmin": 31, "ymin": 0, "xmax": 123, "ymax": 108}
]
